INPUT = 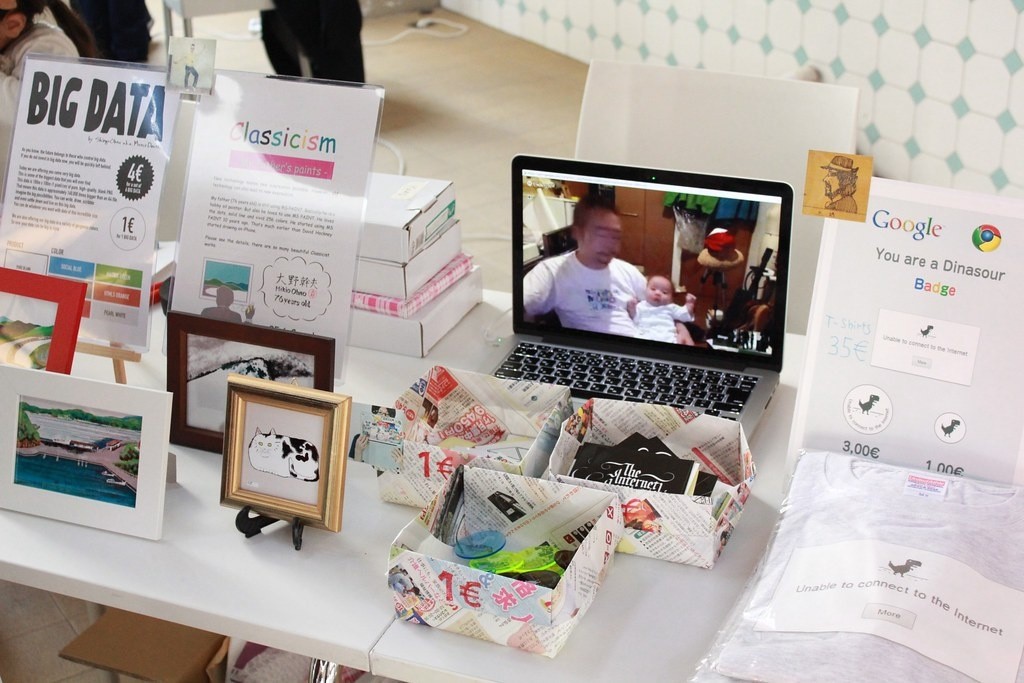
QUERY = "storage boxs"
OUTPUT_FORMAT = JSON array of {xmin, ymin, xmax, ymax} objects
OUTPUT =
[
  {"xmin": 56, "ymin": 606, "xmax": 230, "ymax": 683},
  {"xmin": 344, "ymin": 172, "xmax": 482, "ymax": 358}
]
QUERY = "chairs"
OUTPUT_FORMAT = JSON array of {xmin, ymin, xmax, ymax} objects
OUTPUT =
[{"xmin": 571, "ymin": 61, "xmax": 858, "ymax": 331}]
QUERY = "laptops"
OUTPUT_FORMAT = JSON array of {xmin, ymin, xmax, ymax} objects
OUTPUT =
[{"xmin": 463, "ymin": 154, "xmax": 795, "ymax": 444}]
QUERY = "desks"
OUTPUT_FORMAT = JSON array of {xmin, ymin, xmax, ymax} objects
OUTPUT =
[{"xmin": 0, "ymin": 288, "xmax": 805, "ymax": 683}]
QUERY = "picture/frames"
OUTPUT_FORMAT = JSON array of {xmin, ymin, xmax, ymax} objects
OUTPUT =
[
  {"xmin": 162, "ymin": 308, "xmax": 335, "ymax": 454},
  {"xmin": 221, "ymin": 373, "xmax": 352, "ymax": 532},
  {"xmin": 0, "ymin": 364, "xmax": 174, "ymax": 540}
]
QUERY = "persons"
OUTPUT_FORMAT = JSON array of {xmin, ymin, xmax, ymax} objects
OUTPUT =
[
  {"xmin": 348, "ymin": 405, "xmax": 403, "ymax": 468},
  {"xmin": 628, "ymin": 273, "xmax": 697, "ymax": 343},
  {"xmin": 0, "ymin": 0, "xmax": 100, "ymax": 195},
  {"xmin": 174, "ymin": 43, "xmax": 206, "ymax": 92},
  {"xmin": 201, "ymin": 285, "xmax": 255, "ymax": 324},
  {"xmin": 523, "ymin": 194, "xmax": 695, "ymax": 346}
]
